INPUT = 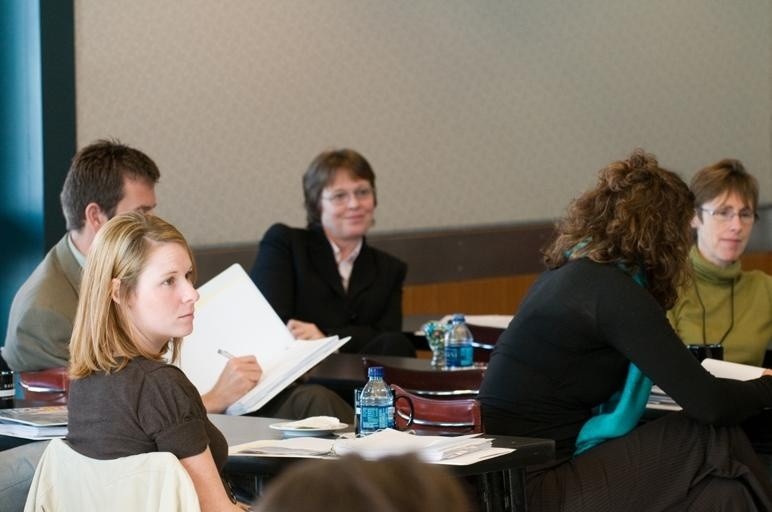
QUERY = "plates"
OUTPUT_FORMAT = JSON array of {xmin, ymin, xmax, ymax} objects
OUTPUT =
[
  {"xmin": 651, "ymin": 384, "xmax": 668, "ymax": 395},
  {"xmin": 268, "ymin": 419, "xmax": 348, "ymax": 438}
]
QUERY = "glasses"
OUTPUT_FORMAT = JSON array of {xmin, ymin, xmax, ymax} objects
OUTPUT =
[
  {"xmin": 698, "ymin": 206, "xmax": 755, "ymax": 225},
  {"xmin": 321, "ymin": 189, "xmax": 374, "ymax": 205}
]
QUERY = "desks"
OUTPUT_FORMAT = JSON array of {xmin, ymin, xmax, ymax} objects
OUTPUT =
[
  {"xmin": 302, "ymin": 352, "xmax": 683, "ymax": 413},
  {"xmin": 207, "ymin": 412, "xmax": 557, "ymax": 512}
]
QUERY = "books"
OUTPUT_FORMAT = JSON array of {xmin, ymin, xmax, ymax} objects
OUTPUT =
[{"xmin": 158, "ymin": 261, "xmax": 356, "ymax": 413}]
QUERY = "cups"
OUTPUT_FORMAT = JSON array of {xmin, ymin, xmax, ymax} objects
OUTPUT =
[
  {"xmin": 349, "ymin": 388, "xmax": 415, "ymax": 437},
  {"xmin": 688, "ymin": 343, "xmax": 722, "ymax": 362},
  {"xmin": 424, "ymin": 324, "xmax": 453, "ymax": 365}
]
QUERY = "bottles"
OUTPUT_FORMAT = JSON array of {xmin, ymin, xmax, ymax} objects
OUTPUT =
[
  {"xmin": 446, "ymin": 314, "xmax": 475, "ymax": 372},
  {"xmin": 360, "ymin": 365, "xmax": 395, "ymax": 438}
]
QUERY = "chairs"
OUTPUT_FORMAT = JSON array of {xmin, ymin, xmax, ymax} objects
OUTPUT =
[
  {"xmin": 390, "ymin": 382, "xmax": 482, "ymax": 433},
  {"xmin": 12, "ymin": 366, "xmax": 68, "ymax": 406},
  {"xmin": 465, "ymin": 323, "xmax": 506, "ymax": 362},
  {"xmin": 23, "ymin": 437, "xmax": 202, "ymax": 512}
]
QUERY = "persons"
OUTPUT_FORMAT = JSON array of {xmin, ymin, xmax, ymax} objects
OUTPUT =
[
  {"xmin": 253, "ymin": 449, "xmax": 473, "ymax": 511},
  {"xmin": 2, "ymin": 138, "xmax": 355, "ymax": 425},
  {"xmin": 664, "ymin": 155, "xmax": 772, "ymax": 367},
  {"xmin": 1, "ymin": 438, "xmax": 52, "ymax": 511},
  {"xmin": 249, "ymin": 147, "xmax": 418, "ymax": 358},
  {"xmin": 455, "ymin": 146, "xmax": 772, "ymax": 511},
  {"xmin": 62, "ymin": 209, "xmax": 253, "ymax": 512}
]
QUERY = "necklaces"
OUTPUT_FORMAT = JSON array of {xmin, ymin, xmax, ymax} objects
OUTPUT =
[{"xmin": 688, "ymin": 258, "xmax": 735, "ymax": 344}]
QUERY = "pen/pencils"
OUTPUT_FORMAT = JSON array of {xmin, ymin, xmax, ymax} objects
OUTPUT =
[{"xmin": 217, "ymin": 349, "xmax": 236, "ymax": 359}]
